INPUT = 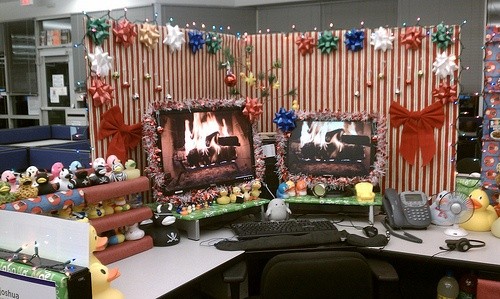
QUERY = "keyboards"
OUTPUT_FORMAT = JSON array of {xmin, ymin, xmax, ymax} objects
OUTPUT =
[{"xmin": 230, "ymin": 220, "xmax": 338, "ymax": 240}]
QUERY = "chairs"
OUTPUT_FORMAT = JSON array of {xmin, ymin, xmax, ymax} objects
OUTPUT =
[{"xmin": 221, "ymin": 253, "xmax": 398, "ymax": 299}]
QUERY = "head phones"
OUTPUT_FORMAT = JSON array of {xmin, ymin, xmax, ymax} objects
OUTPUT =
[{"xmin": 445, "ymin": 238, "xmax": 486, "ymax": 252}]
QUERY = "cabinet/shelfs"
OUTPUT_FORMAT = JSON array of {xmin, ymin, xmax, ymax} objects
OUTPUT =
[
  {"xmin": 0, "ymin": 166, "xmax": 153, "ymax": 266},
  {"xmin": 0, "ymin": 12, "xmax": 88, "ymax": 127}
]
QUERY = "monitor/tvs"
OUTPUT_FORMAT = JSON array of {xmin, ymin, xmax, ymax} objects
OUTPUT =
[
  {"xmin": 153, "ymin": 105, "xmax": 257, "ymax": 197},
  {"xmin": 284, "ymin": 118, "xmax": 377, "ymax": 198}
]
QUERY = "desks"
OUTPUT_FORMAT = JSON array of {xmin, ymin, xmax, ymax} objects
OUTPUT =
[{"xmin": 101, "ymin": 213, "xmax": 500, "ymax": 299}]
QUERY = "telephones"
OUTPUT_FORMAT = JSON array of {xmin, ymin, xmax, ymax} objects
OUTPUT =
[{"xmin": 383, "ymin": 188, "xmax": 432, "ymax": 229}]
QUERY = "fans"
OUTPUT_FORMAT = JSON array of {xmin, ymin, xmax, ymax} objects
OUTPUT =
[{"xmin": 438, "ymin": 191, "xmax": 475, "ymax": 237}]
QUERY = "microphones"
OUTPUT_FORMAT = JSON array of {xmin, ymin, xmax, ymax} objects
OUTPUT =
[{"xmin": 439, "ymin": 247, "xmax": 454, "ymax": 251}]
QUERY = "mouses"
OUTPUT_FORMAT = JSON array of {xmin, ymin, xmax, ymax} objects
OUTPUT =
[{"xmin": 362, "ymin": 226, "xmax": 378, "ymax": 237}]
